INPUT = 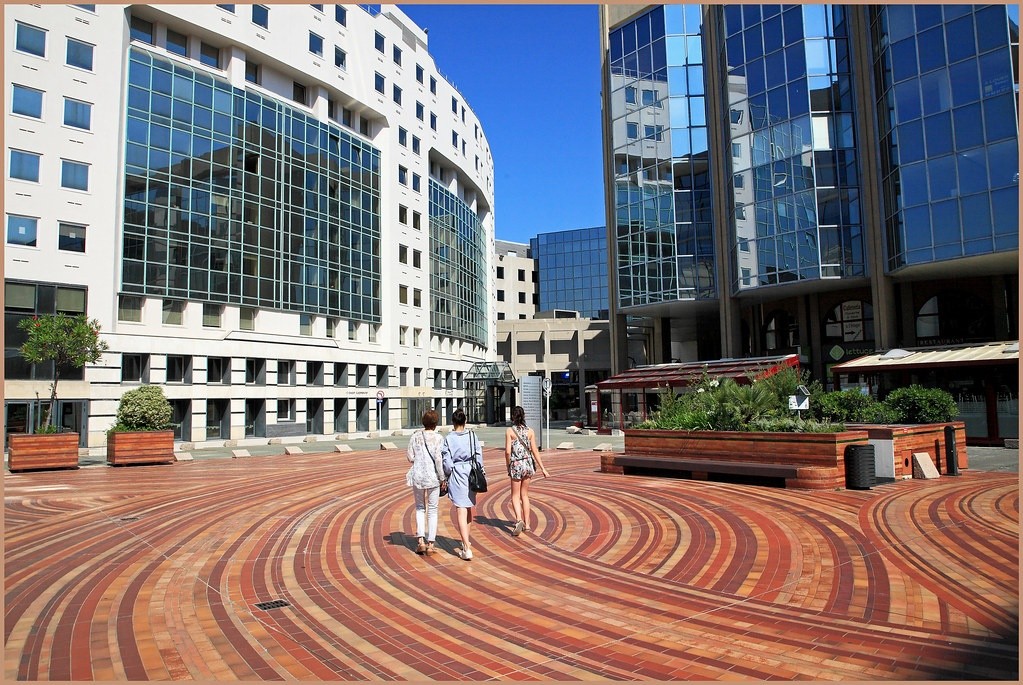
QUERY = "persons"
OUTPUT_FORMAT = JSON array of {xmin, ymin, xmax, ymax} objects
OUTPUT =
[
  {"xmin": 441, "ymin": 408, "xmax": 488, "ymax": 560},
  {"xmin": 405, "ymin": 410, "xmax": 448, "ymax": 556},
  {"xmin": 505, "ymin": 406, "xmax": 551, "ymax": 536}
]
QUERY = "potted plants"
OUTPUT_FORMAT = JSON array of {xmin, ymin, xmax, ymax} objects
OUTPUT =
[
  {"xmin": 8, "ymin": 312, "xmax": 110, "ymax": 472},
  {"xmin": 102, "ymin": 383, "xmax": 175, "ymax": 467}
]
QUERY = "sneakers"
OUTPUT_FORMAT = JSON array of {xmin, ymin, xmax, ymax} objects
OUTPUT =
[
  {"xmin": 459, "ymin": 542, "xmax": 472, "ymax": 548},
  {"xmin": 458, "ymin": 549, "xmax": 473, "ymax": 559}
]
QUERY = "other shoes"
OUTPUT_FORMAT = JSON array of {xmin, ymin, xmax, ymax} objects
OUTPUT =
[
  {"xmin": 512, "ymin": 520, "xmax": 524, "ymax": 535},
  {"xmin": 427, "ymin": 547, "xmax": 435, "ymax": 556},
  {"xmin": 414, "ymin": 546, "xmax": 426, "ymax": 554},
  {"xmin": 513, "ymin": 523, "xmax": 530, "ymax": 531}
]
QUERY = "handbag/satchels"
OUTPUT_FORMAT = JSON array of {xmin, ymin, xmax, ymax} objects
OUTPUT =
[
  {"xmin": 531, "ymin": 456, "xmax": 536, "ymax": 471},
  {"xmin": 468, "ymin": 463, "xmax": 488, "ymax": 493},
  {"xmin": 439, "ymin": 477, "xmax": 448, "ymax": 496}
]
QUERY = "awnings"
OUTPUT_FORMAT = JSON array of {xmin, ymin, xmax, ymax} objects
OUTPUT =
[{"xmin": 829, "ymin": 340, "xmax": 1019, "ymax": 373}]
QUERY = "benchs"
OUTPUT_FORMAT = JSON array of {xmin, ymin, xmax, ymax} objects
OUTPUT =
[{"xmin": 600, "ymin": 453, "xmax": 839, "ymax": 491}]
